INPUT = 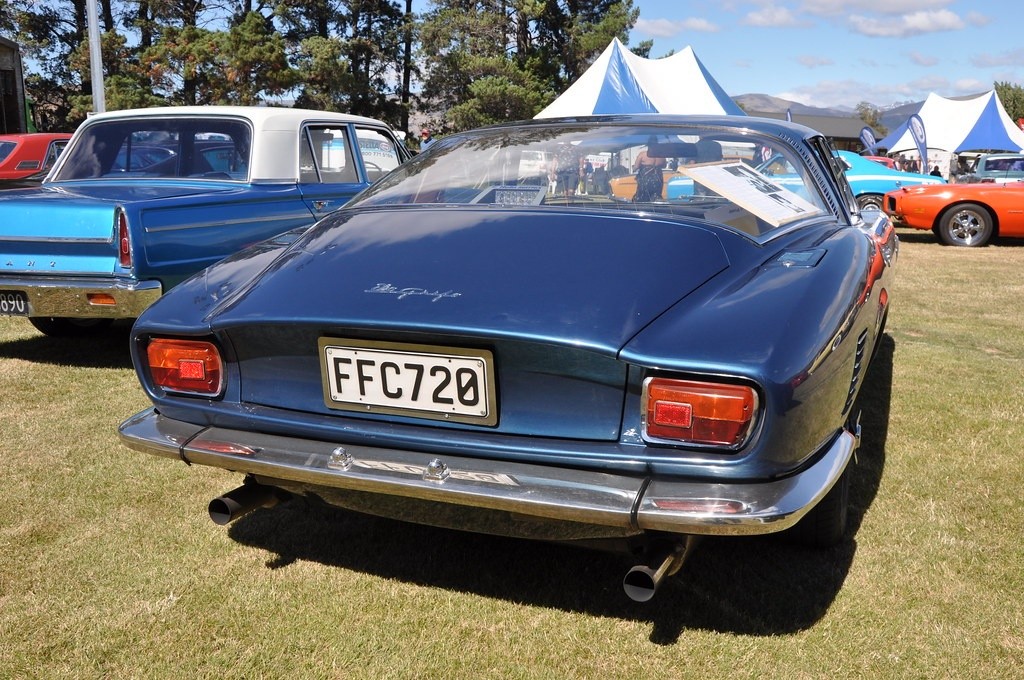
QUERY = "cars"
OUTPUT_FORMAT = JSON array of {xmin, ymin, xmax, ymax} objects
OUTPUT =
[
  {"xmin": 109, "ymin": 138, "xmax": 248, "ymax": 177},
  {"xmin": 665, "ymin": 148, "xmax": 949, "ymax": 224},
  {"xmin": 863, "ymin": 155, "xmax": 899, "ymax": 173},
  {"xmin": 0, "ymin": 101, "xmax": 425, "ymax": 345},
  {"xmin": 110, "ymin": 114, "xmax": 899, "ymax": 604},
  {"xmin": 1, "ymin": 132, "xmax": 74, "ymax": 180},
  {"xmin": 963, "ymin": 154, "xmax": 1024, "ymax": 186},
  {"xmin": 605, "ymin": 154, "xmax": 788, "ymax": 200}
]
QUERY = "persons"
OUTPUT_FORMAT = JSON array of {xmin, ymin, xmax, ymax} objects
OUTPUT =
[
  {"xmin": 690, "ymin": 135, "xmax": 722, "ymax": 196},
  {"xmin": 632, "ymin": 135, "xmax": 667, "ymax": 202},
  {"xmin": 893, "ymin": 153, "xmax": 921, "ymax": 173},
  {"xmin": 549, "ymin": 140, "xmax": 592, "ymax": 197},
  {"xmin": 420, "ymin": 129, "xmax": 437, "ymax": 152},
  {"xmin": 753, "ymin": 143, "xmax": 772, "ymax": 174},
  {"xmin": 930, "ymin": 166, "xmax": 942, "ymax": 177}
]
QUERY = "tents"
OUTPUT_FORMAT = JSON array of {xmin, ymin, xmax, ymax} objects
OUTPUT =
[
  {"xmin": 532, "ymin": 37, "xmax": 755, "ymax": 148},
  {"xmin": 859, "ymin": 89, "xmax": 1024, "ymax": 155}
]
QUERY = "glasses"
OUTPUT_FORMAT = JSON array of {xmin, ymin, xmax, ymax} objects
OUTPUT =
[{"xmin": 422, "ymin": 133, "xmax": 428, "ymax": 136}]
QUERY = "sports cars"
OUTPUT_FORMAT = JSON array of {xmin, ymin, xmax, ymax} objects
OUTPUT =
[{"xmin": 884, "ymin": 177, "xmax": 1024, "ymax": 248}]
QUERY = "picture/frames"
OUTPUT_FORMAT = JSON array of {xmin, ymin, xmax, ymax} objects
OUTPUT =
[{"xmin": 677, "ymin": 159, "xmax": 824, "ymax": 229}]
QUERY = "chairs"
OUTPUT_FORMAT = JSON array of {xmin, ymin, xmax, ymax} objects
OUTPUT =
[{"xmin": 438, "ymin": 186, "xmax": 496, "ymax": 204}]
